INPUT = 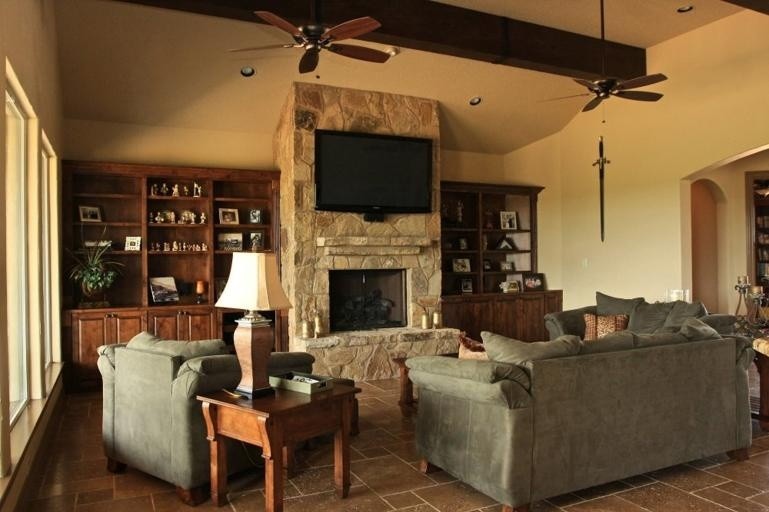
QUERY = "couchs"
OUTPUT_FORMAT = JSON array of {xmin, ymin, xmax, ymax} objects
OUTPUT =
[
  {"xmin": 544, "ymin": 292, "xmax": 738, "ymax": 340},
  {"xmin": 404, "ymin": 316, "xmax": 755, "ymax": 512},
  {"xmin": 96, "ymin": 331, "xmax": 315, "ymax": 506}
]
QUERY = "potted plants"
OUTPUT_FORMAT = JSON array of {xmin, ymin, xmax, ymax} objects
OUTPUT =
[{"xmin": 64, "ymin": 223, "xmax": 125, "ymax": 309}]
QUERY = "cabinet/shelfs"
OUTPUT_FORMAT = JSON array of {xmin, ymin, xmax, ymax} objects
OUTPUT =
[
  {"xmin": 440, "ymin": 180, "xmax": 563, "ymax": 343},
  {"xmin": 60, "ymin": 159, "xmax": 281, "ymax": 377}
]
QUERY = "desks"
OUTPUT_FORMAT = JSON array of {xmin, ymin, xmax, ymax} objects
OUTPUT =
[{"xmin": 196, "ymin": 386, "xmax": 362, "ymax": 512}]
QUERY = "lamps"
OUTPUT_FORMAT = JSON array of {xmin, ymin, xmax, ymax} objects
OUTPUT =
[{"xmin": 214, "ymin": 252, "xmax": 294, "ymax": 400}]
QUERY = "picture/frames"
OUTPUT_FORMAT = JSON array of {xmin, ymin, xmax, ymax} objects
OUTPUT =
[
  {"xmin": 218, "ymin": 207, "xmax": 239, "ymax": 225},
  {"xmin": 452, "ymin": 255, "xmax": 472, "ymax": 273},
  {"xmin": 522, "ymin": 271, "xmax": 545, "ymax": 293},
  {"xmin": 150, "ymin": 276, "xmax": 180, "ymax": 303},
  {"xmin": 500, "ymin": 211, "xmax": 516, "ymax": 230},
  {"xmin": 247, "ymin": 230, "xmax": 266, "ymax": 253},
  {"xmin": 216, "ymin": 231, "xmax": 245, "ymax": 251},
  {"xmin": 249, "ymin": 207, "xmax": 263, "ymax": 223},
  {"xmin": 79, "ymin": 206, "xmax": 103, "ymax": 222}
]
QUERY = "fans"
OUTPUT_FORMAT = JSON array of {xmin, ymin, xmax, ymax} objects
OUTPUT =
[
  {"xmin": 538, "ymin": 1, "xmax": 668, "ymax": 113},
  {"xmin": 225, "ymin": 11, "xmax": 390, "ymax": 73}
]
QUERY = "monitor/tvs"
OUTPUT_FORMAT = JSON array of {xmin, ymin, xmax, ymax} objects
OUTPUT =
[{"xmin": 314, "ymin": 130, "xmax": 433, "ymax": 213}]
